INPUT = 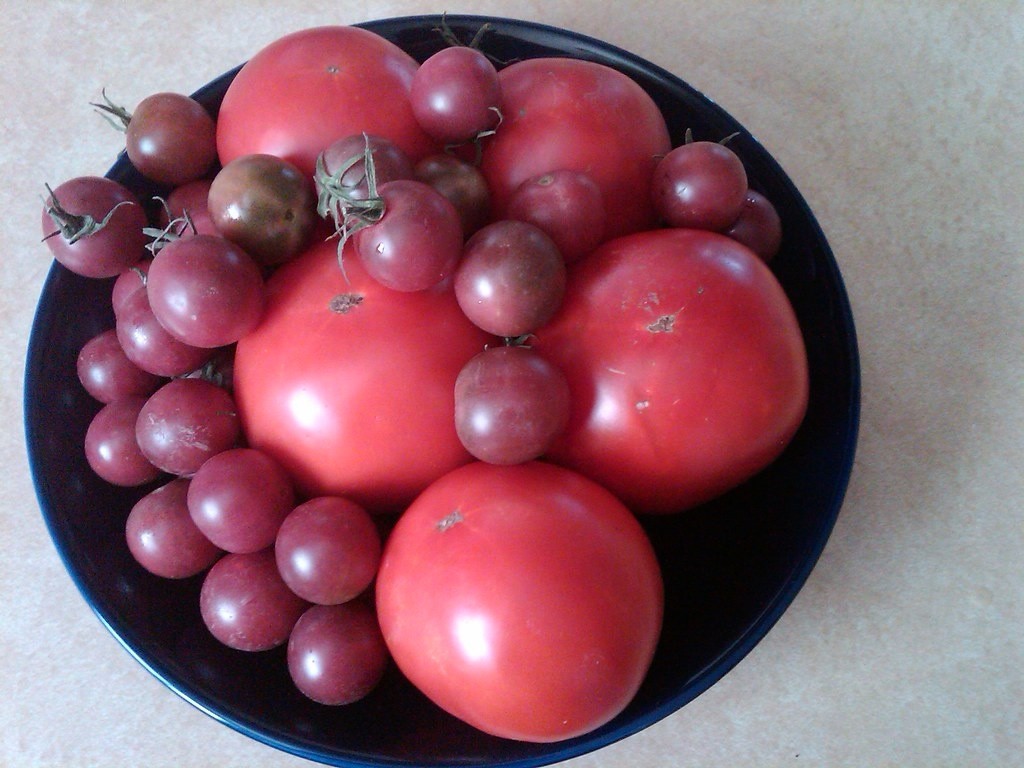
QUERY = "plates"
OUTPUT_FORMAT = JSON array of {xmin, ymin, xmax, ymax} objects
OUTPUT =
[{"xmin": 22, "ymin": 10, "xmax": 863, "ymax": 768}]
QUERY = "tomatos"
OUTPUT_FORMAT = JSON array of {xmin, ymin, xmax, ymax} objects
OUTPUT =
[{"xmin": 35, "ymin": 26, "xmax": 808, "ymax": 743}]
